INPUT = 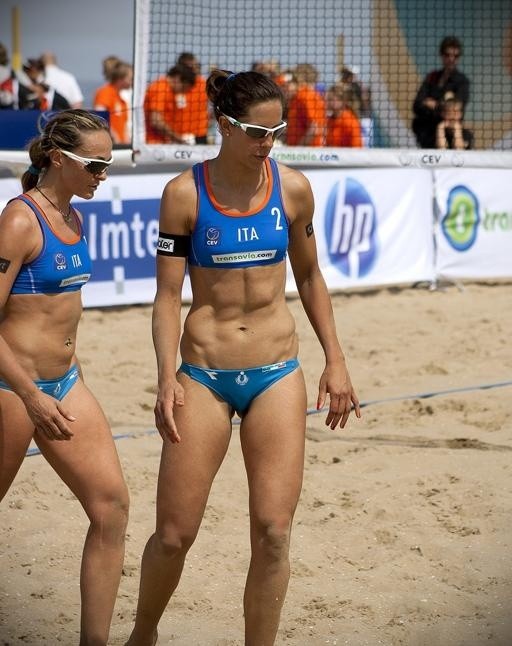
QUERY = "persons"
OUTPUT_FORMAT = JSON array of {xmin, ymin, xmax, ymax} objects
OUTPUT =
[
  {"xmin": 411, "ymin": 34, "xmax": 471, "ymax": 148},
  {"xmin": 94, "ymin": 52, "xmax": 372, "ymax": 148},
  {"xmin": 435, "ymin": 99, "xmax": 474, "ymax": 150},
  {"xmin": 1, "ymin": 108, "xmax": 130, "ymax": 646},
  {"xmin": 2, "ymin": 46, "xmax": 84, "ymax": 111},
  {"xmin": 127, "ymin": 66, "xmax": 362, "ymax": 644}
]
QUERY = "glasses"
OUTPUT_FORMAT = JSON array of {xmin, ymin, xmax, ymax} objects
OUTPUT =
[
  {"xmin": 216, "ymin": 105, "xmax": 289, "ymax": 141},
  {"xmin": 55, "ymin": 147, "xmax": 113, "ymax": 176}
]
